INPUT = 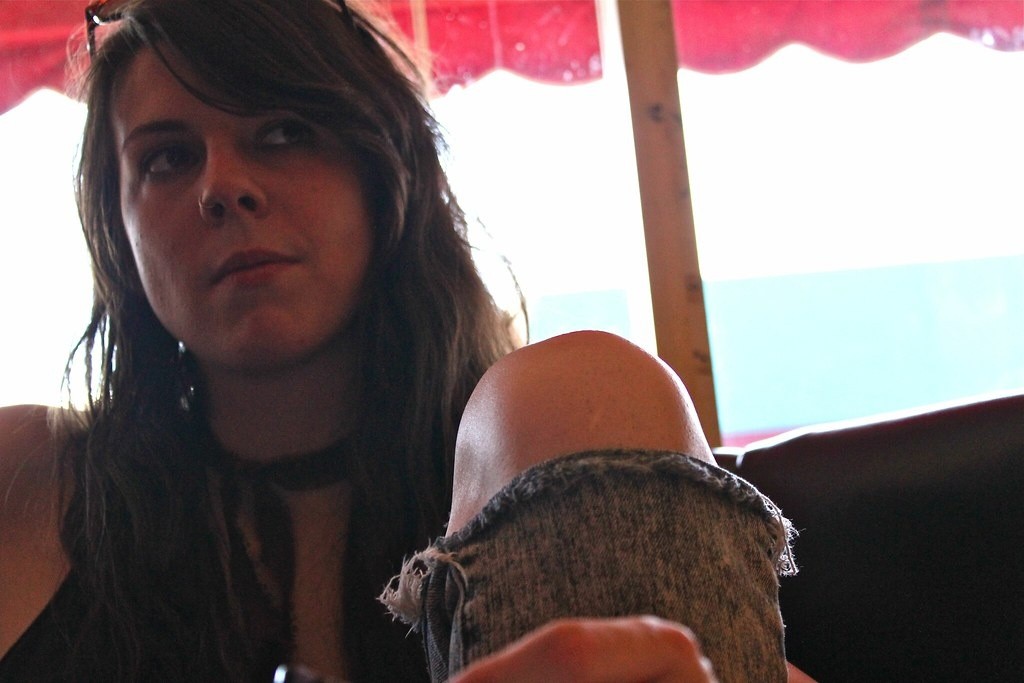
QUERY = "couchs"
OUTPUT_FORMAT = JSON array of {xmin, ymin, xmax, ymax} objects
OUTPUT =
[{"xmin": 714, "ymin": 393, "xmax": 1023, "ymax": 683}]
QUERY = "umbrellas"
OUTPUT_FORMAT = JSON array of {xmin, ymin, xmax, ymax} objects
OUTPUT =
[{"xmin": 0, "ymin": 0, "xmax": 1024, "ymax": 479}]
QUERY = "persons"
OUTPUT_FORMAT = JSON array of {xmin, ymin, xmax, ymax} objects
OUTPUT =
[{"xmin": 1, "ymin": 0, "xmax": 811, "ymax": 681}]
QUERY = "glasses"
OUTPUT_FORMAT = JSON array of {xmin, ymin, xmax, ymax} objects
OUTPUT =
[{"xmin": 84, "ymin": 0, "xmax": 147, "ymax": 63}]
